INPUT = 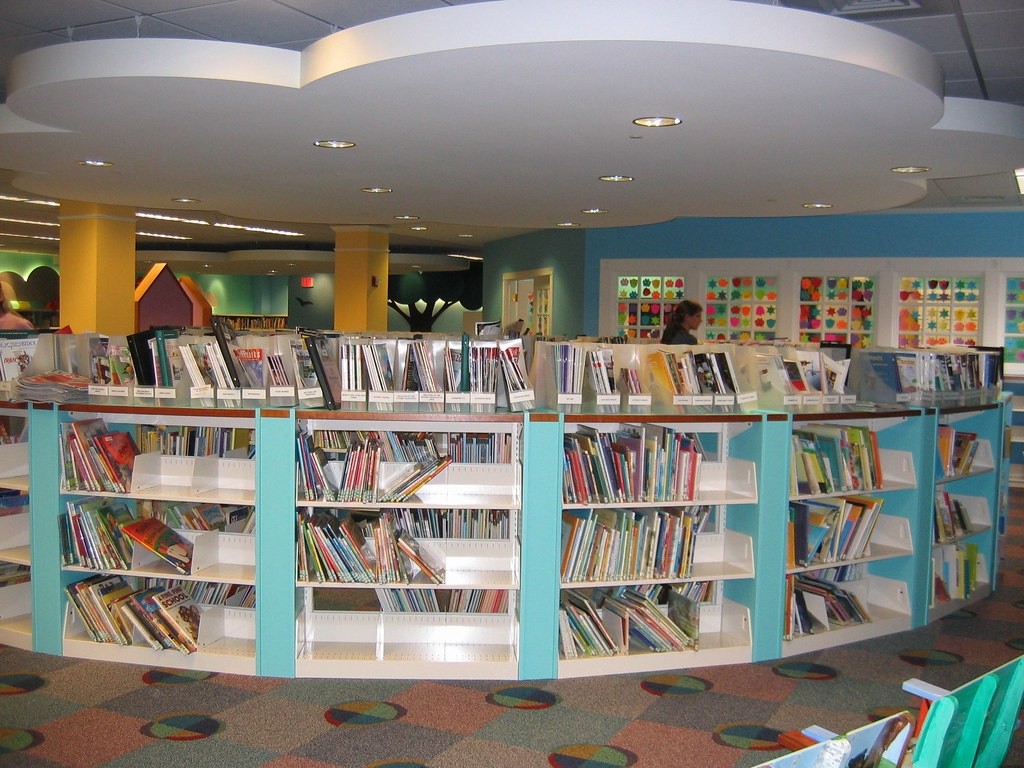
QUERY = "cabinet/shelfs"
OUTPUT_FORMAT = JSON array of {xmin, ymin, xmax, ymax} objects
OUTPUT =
[
  {"xmin": 0, "ymin": 390, "xmax": 1014, "ymax": 682},
  {"xmin": 1004, "ymin": 374, "xmax": 1024, "ymax": 489},
  {"xmin": 212, "ymin": 314, "xmax": 288, "ymax": 332}
]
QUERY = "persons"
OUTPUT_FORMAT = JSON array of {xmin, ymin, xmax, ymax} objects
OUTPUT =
[{"xmin": 659, "ymin": 300, "xmax": 704, "ymax": 344}]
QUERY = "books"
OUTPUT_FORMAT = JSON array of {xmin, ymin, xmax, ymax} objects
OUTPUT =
[
  {"xmin": 296, "ymin": 507, "xmax": 512, "ymax": 583},
  {"xmin": 374, "ymin": 589, "xmax": 509, "ymax": 613},
  {"xmin": 0, "ymin": 414, "xmax": 32, "ymax": 588},
  {"xmin": 785, "ymin": 563, "xmax": 872, "ymax": 641},
  {"xmin": 861, "ymin": 345, "xmax": 1006, "ymax": 399},
  {"xmin": 546, "ymin": 344, "xmax": 741, "ymax": 396},
  {"xmin": 564, "ymin": 506, "xmax": 712, "ymax": 578},
  {"xmin": 789, "ymin": 496, "xmax": 884, "ymax": 568},
  {"xmin": 563, "ymin": 423, "xmax": 707, "ymax": 503},
  {"xmin": 935, "ymin": 423, "xmax": 979, "ymax": 479},
  {"xmin": 476, "ymin": 319, "xmax": 852, "ymax": 347},
  {"xmin": 60, "ymin": 498, "xmax": 255, "ymax": 573},
  {"xmin": 298, "ymin": 432, "xmax": 516, "ymax": 504},
  {"xmin": 217, "ymin": 314, "xmax": 287, "ymax": 333},
  {"xmin": 936, "ymin": 542, "xmax": 979, "ymax": 604},
  {"xmin": 0, "ymin": 327, "xmax": 538, "ymax": 409},
  {"xmin": 756, "ymin": 350, "xmax": 851, "ymax": 399},
  {"xmin": 63, "ymin": 575, "xmax": 258, "ymax": 655},
  {"xmin": 791, "ymin": 424, "xmax": 883, "ymax": 494},
  {"xmin": 934, "ymin": 491, "xmax": 974, "ymax": 543},
  {"xmin": 560, "ymin": 582, "xmax": 713, "ymax": 657},
  {"xmin": 59, "ymin": 419, "xmax": 256, "ymax": 492}
]
